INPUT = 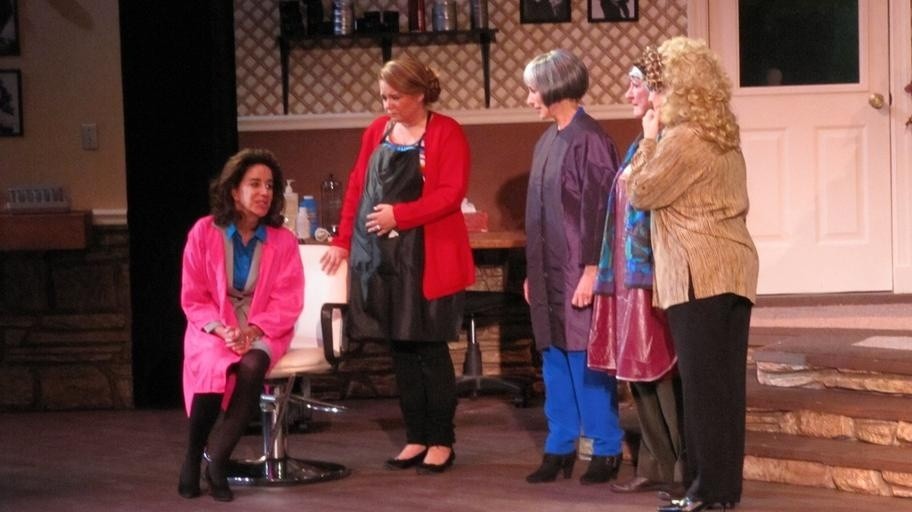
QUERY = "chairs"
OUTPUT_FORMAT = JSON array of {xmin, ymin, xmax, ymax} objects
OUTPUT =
[
  {"xmin": 224, "ymin": 243, "xmax": 351, "ymax": 487},
  {"xmin": 456, "ymin": 247, "xmax": 534, "ymax": 406}
]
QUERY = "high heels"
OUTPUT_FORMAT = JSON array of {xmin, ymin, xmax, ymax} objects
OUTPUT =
[
  {"xmin": 664, "ymin": 492, "xmax": 735, "ymax": 509},
  {"xmin": 581, "ymin": 454, "xmax": 620, "ymax": 484},
  {"xmin": 205, "ymin": 465, "xmax": 235, "ymax": 502},
  {"xmin": 656, "ymin": 496, "xmax": 727, "ymax": 512},
  {"xmin": 526, "ymin": 448, "xmax": 576, "ymax": 484},
  {"xmin": 177, "ymin": 464, "xmax": 201, "ymax": 499}
]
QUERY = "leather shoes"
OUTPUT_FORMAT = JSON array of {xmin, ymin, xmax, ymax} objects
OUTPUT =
[{"xmin": 611, "ymin": 477, "xmax": 675, "ymax": 492}]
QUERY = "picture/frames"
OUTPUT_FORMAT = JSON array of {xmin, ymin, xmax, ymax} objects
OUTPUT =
[
  {"xmin": 519, "ymin": 0, "xmax": 572, "ymax": 24},
  {"xmin": 0, "ymin": 5, "xmax": 20, "ymax": 59},
  {"xmin": 588, "ymin": 0, "xmax": 639, "ymax": 23},
  {"xmin": 0, "ymin": 70, "xmax": 24, "ymax": 136}
]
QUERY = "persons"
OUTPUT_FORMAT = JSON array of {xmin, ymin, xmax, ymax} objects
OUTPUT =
[
  {"xmin": 320, "ymin": 59, "xmax": 477, "ymax": 476},
  {"xmin": 587, "ymin": 58, "xmax": 693, "ymax": 501},
  {"xmin": 628, "ymin": 36, "xmax": 760, "ymax": 512},
  {"xmin": 178, "ymin": 148, "xmax": 304, "ymax": 503},
  {"xmin": 523, "ymin": 48, "xmax": 622, "ymax": 485}
]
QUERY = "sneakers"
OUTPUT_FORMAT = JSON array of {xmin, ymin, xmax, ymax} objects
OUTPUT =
[
  {"xmin": 416, "ymin": 447, "xmax": 456, "ymax": 475},
  {"xmin": 384, "ymin": 447, "xmax": 428, "ymax": 470}
]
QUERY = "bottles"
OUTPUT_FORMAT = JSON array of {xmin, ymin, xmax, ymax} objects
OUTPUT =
[
  {"xmin": 320, "ymin": 170, "xmax": 346, "ymax": 238},
  {"xmin": 294, "ymin": 205, "xmax": 312, "ymax": 240},
  {"xmin": 407, "ymin": 0, "xmax": 416, "ymax": 31},
  {"xmin": 299, "ymin": 193, "xmax": 319, "ymax": 239},
  {"xmin": 430, "ymin": 0, "xmax": 456, "ymax": 31},
  {"xmin": 468, "ymin": 1, "xmax": 488, "ymax": 29},
  {"xmin": 332, "ymin": 0, "xmax": 355, "ymax": 37}
]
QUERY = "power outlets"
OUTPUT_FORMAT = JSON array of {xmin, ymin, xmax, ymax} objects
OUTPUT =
[{"xmin": 83, "ymin": 123, "xmax": 98, "ymax": 151}]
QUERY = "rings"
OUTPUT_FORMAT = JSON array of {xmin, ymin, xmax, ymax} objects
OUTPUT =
[{"xmin": 376, "ymin": 225, "xmax": 381, "ymax": 231}]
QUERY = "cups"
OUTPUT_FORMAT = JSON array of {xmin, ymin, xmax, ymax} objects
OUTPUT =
[
  {"xmin": 383, "ymin": 11, "xmax": 399, "ymax": 33},
  {"xmin": 363, "ymin": 10, "xmax": 381, "ymax": 30}
]
